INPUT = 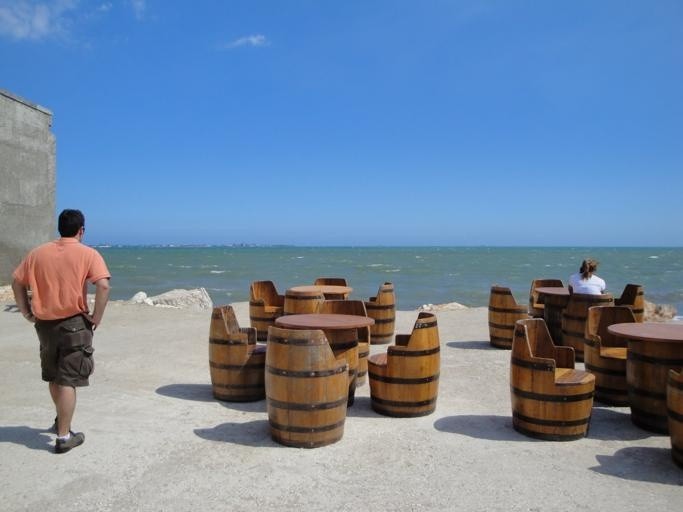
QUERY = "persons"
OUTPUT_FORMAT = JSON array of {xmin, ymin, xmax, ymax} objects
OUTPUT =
[
  {"xmin": 9, "ymin": 209, "xmax": 112, "ymax": 454},
  {"xmin": 567, "ymin": 258, "xmax": 607, "ymax": 296}
]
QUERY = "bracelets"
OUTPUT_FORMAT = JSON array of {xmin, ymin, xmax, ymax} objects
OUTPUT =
[{"xmin": 22, "ymin": 312, "xmax": 31, "ymax": 318}]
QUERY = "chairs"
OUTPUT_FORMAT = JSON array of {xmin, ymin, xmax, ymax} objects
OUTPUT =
[
  {"xmin": 666, "ymin": 368, "xmax": 680, "ymax": 464},
  {"xmin": 584, "ymin": 307, "xmax": 638, "ymax": 407},
  {"xmin": 511, "ymin": 318, "xmax": 596, "ymax": 441},
  {"xmin": 264, "ymin": 326, "xmax": 351, "ymax": 449},
  {"xmin": 212, "ymin": 305, "xmax": 267, "ymax": 402},
  {"xmin": 210, "ymin": 278, "xmax": 644, "ymax": 350},
  {"xmin": 367, "ymin": 312, "xmax": 441, "ymax": 418}
]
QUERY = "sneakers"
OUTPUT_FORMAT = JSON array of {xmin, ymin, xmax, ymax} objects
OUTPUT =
[{"xmin": 52, "ymin": 415, "xmax": 85, "ymax": 455}]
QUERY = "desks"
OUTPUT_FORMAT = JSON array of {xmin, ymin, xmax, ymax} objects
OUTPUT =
[
  {"xmin": 274, "ymin": 313, "xmax": 375, "ymax": 408},
  {"xmin": 607, "ymin": 322, "xmax": 680, "ymax": 434}
]
